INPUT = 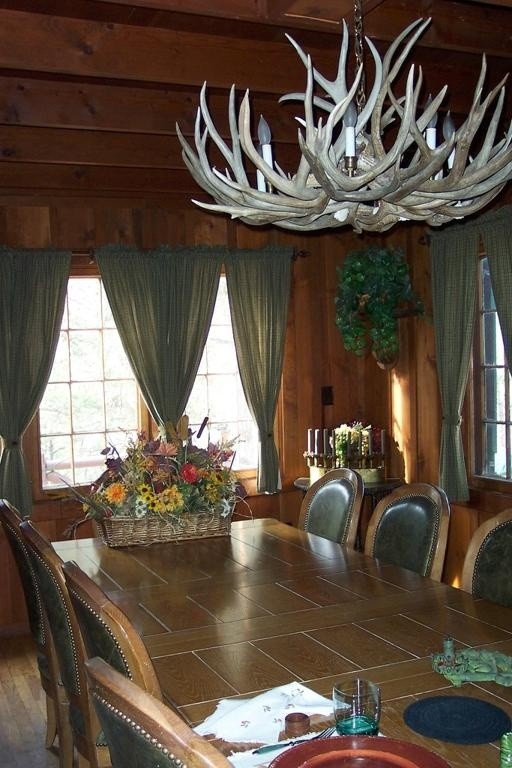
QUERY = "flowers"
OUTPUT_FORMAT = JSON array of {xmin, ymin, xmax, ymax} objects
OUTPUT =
[
  {"xmin": 83, "ymin": 436, "xmax": 234, "ymax": 517},
  {"xmin": 330, "ymin": 421, "xmax": 375, "ymax": 450}
]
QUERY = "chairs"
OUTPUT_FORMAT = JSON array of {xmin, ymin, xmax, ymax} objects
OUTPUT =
[
  {"xmin": 365, "ymin": 479, "xmax": 452, "ymax": 574},
  {"xmin": 60, "ymin": 561, "xmax": 169, "ymax": 768},
  {"xmin": 297, "ymin": 469, "xmax": 362, "ymax": 547},
  {"xmin": 0, "ymin": 500, "xmax": 72, "ymax": 768},
  {"xmin": 88, "ymin": 656, "xmax": 239, "ymax": 768},
  {"xmin": 462, "ymin": 508, "xmax": 510, "ymax": 605},
  {"xmin": 21, "ymin": 516, "xmax": 137, "ymax": 767}
]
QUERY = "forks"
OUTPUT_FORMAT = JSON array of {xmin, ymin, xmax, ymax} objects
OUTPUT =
[{"xmin": 253, "ymin": 727, "xmax": 336, "ymax": 754}]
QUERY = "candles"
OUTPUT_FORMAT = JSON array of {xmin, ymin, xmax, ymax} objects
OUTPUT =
[{"xmin": 306, "ymin": 427, "xmax": 387, "ymax": 454}]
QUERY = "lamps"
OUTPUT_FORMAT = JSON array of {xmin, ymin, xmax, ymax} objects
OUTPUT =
[{"xmin": 172, "ymin": 5, "xmax": 512, "ymax": 236}]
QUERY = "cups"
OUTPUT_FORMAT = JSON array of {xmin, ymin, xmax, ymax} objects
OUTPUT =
[
  {"xmin": 332, "ymin": 678, "xmax": 380, "ymax": 737},
  {"xmin": 284, "ymin": 713, "xmax": 311, "ymax": 737}
]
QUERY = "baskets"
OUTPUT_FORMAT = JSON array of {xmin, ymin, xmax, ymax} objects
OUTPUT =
[{"xmin": 94, "ymin": 510, "xmax": 234, "ymax": 549}]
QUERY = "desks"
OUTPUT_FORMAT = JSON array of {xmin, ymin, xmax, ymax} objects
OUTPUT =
[{"xmin": 51, "ymin": 517, "xmax": 511, "ymax": 768}]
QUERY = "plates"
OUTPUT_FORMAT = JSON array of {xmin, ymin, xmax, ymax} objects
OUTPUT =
[{"xmin": 269, "ymin": 735, "xmax": 451, "ymax": 768}]
